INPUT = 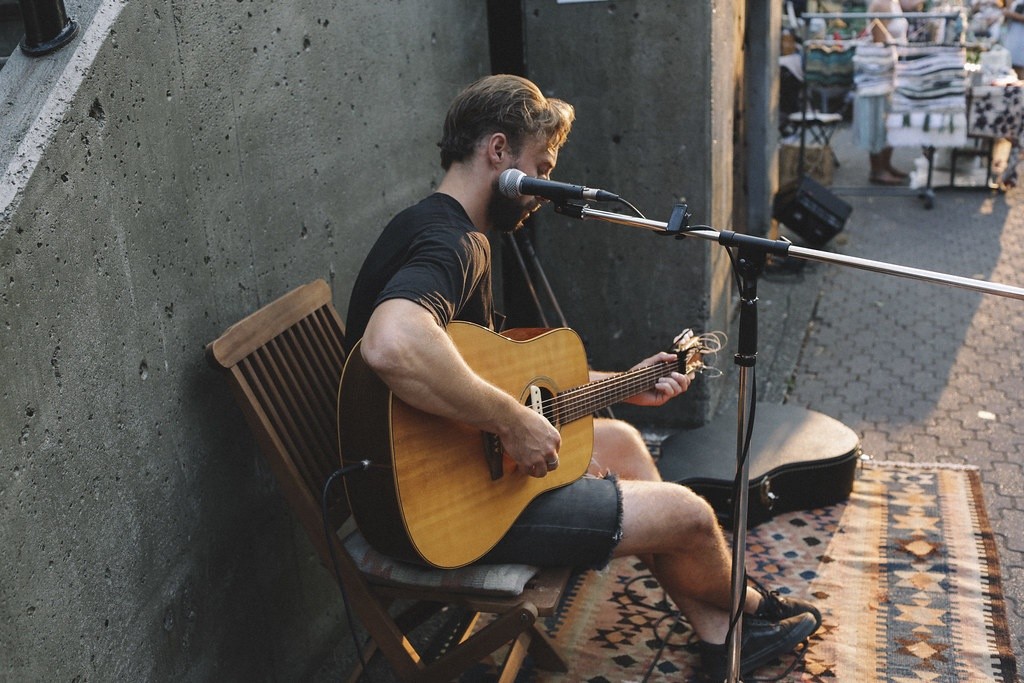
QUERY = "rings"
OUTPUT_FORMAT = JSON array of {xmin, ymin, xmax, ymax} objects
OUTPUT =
[{"xmin": 547, "ymin": 455, "xmax": 559, "ymax": 465}]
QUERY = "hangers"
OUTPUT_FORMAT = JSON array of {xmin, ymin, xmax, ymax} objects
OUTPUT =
[{"xmin": 806, "ymin": 16, "xmax": 961, "ymax": 44}]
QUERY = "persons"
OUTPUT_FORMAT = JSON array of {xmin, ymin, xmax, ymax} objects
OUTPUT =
[
  {"xmin": 345, "ymin": 75, "xmax": 822, "ymax": 683},
  {"xmin": 853, "ymin": 0, "xmax": 1024, "ymax": 185}
]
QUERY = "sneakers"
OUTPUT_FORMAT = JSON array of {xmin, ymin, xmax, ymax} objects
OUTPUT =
[{"xmin": 699, "ymin": 587, "xmax": 821, "ymax": 683}]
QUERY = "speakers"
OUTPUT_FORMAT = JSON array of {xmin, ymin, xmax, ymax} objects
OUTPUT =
[{"xmin": 770, "ymin": 175, "xmax": 852, "ymax": 247}]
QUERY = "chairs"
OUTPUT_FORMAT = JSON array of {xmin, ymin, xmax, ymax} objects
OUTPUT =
[
  {"xmin": 202, "ymin": 278, "xmax": 574, "ymax": 683},
  {"xmin": 778, "ymin": 63, "xmax": 843, "ymax": 169}
]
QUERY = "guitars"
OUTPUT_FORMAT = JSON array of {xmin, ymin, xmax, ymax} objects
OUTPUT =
[{"xmin": 336, "ymin": 321, "xmax": 728, "ymax": 568}]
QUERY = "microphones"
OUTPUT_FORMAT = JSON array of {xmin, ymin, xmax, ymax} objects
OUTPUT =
[{"xmin": 498, "ymin": 168, "xmax": 619, "ymax": 201}]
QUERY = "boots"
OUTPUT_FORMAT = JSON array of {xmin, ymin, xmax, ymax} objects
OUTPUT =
[{"xmin": 868, "ymin": 146, "xmax": 908, "ymax": 185}]
